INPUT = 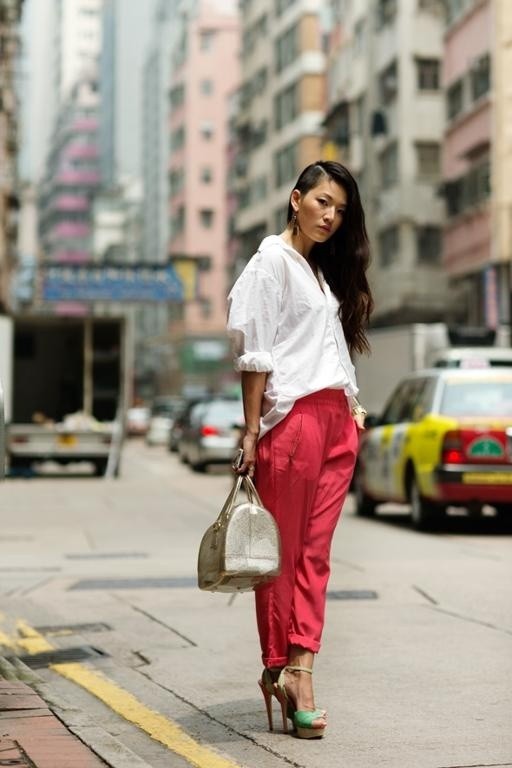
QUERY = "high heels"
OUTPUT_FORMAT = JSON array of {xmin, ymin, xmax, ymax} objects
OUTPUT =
[
  {"xmin": 258, "ymin": 666, "xmax": 329, "ymax": 730},
  {"xmin": 273, "ymin": 663, "xmax": 327, "ymax": 739}
]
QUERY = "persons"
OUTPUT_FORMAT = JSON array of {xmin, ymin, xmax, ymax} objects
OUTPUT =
[{"xmin": 226, "ymin": 159, "xmax": 375, "ymax": 739}]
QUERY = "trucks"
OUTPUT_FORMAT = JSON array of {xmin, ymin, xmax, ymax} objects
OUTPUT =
[{"xmin": 0, "ymin": 311, "xmax": 131, "ymax": 478}]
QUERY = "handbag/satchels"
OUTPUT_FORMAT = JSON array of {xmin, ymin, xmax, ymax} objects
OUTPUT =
[{"xmin": 197, "ymin": 472, "xmax": 283, "ymax": 594}]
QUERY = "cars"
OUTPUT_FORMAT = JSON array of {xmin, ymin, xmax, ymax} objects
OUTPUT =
[
  {"xmin": 355, "ymin": 365, "xmax": 512, "ymax": 530},
  {"xmin": 147, "ymin": 396, "xmax": 248, "ymax": 471}
]
